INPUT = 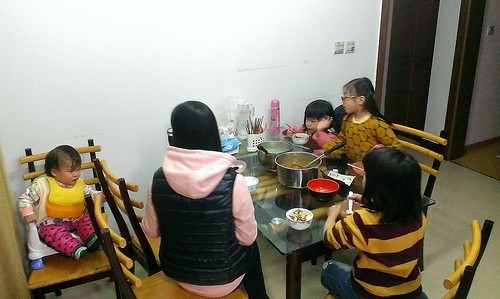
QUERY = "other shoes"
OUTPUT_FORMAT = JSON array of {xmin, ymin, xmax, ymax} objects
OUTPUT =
[
  {"xmin": 72, "ymin": 245, "xmax": 87, "ymax": 260},
  {"xmin": 87, "ymin": 233, "xmax": 101, "ymax": 250}
]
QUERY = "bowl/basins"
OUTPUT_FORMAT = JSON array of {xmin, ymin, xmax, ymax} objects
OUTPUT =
[
  {"xmin": 292, "ymin": 133, "xmax": 310, "ymax": 145},
  {"xmin": 244, "ymin": 177, "xmax": 260, "ymax": 192},
  {"xmin": 307, "ymin": 179, "xmax": 339, "ymax": 202},
  {"xmin": 286, "ymin": 208, "xmax": 313, "ymax": 230},
  {"xmin": 337, "ymin": 200, "xmax": 361, "ymax": 219},
  {"xmin": 352, "ymin": 162, "xmax": 364, "ymax": 177}
]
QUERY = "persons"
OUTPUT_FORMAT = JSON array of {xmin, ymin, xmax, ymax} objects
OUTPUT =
[
  {"xmin": 282, "ymin": 99, "xmax": 338, "ymax": 149},
  {"xmin": 312, "ymin": 76, "xmax": 403, "ymax": 163},
  {"xmin": 18, "ymin": 145, "xmax": 114, "ymax": 261},
  {"xmin": 321, "ymin": 147, "xmax": 428, "ymax": 299},
  {"xmin": 141, "ymin": 101, "xmax": 270, "ymax": 298}
]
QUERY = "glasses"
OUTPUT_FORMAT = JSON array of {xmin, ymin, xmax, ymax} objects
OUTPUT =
[
  {"xmin": 167, "ymin": 128, "xmax": 174, "ymax": 137},
  {"xmin": 341, "ymin": 96, "xmax": 355, "ymax": 100}
]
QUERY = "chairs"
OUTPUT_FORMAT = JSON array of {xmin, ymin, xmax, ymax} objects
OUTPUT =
[
  {"xmin": 324, "ymin": 220, "xmax": 494, "ymax": 299},
  {"xmin": 19, "ymin": 139, "xmax": 113, "ymax": 299},
  {"xmin": 85, "ymin": 193, "xmax": 250, "ymax": 299},
  {"xmin": 325, "ymin": 123, "xmax": 449, "ymax": 270},
  {"xmin": 91, "ymin": 157, "xmax": 161, "ymax": 277}
]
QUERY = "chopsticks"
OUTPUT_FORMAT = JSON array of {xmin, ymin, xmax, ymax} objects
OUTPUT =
[{"xmin": 245, "ymin": 114, "xmax": 264, "ymax": 134}]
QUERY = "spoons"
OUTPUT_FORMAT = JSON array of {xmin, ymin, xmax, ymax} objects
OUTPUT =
[{"xmin": 346, "ymin": 191, "xmax": 354, "ymax": 215}]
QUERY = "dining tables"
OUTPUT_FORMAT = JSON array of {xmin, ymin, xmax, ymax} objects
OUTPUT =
[{"xmin": 230, "ymin": 126, "xmax": 435, "ymax": 299}]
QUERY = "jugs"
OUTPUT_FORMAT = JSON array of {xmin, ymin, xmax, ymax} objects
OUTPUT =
[{"xmin": 236, "ymin": 103, "xmax": 256, "ymax": 140}]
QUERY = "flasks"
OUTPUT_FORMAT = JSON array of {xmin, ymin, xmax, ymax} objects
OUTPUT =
[{"xmin": 270, "ymin": 99, "xmax": 280, "ymax": 136}]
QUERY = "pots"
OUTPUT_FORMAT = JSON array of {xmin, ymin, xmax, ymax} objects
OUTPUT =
[
  {"xmin": 257, "ymin": 141, "xmax": 293, "ymax": 171},
  {"xmin": 274, "ymin": 152, "xmax": 323, "ymax": 189}
]
